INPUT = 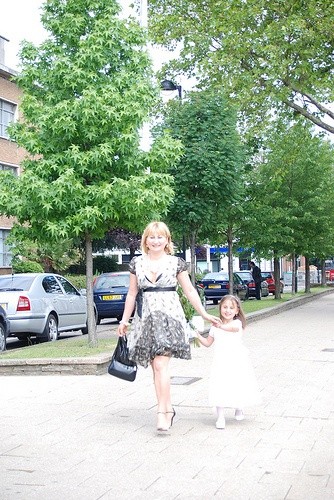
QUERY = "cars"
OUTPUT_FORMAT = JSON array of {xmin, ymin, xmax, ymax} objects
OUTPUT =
[
  {"xmin": 0, "ymin": 306, "xmax": 8, "ymax": 352},
  {"xmin": 199, "ymin": 272, "xmax": 248, "ymax": 304},
  {"xmin": 261, "ymin": 271, "xmax": 285, "ymax": 294},
  {"xmin": 93, "ymin": 271, "xmax": 131, "ymax": 325},
  {"xmin": 0, "ymin": 272, "xmax": 98, "ymax": 352},
  {"xmin": 235, "ymin": 272, "xmax": 269, "ymax": 296}
]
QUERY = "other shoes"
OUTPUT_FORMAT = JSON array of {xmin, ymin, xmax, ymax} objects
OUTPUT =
[
  {"xmin": 215, "ymin": 417, "xmax": 225, "ymax": 429},
  {"xmin": 155, "ymin": 407, "xmax": 177, "ymax": 434},
  {"xmin": 234, "ymin": 409, "xmax": 245, "ymax": 420}
]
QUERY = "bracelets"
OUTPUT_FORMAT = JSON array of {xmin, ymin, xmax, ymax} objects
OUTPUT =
[{"xmin": 120, "ymin": 321, "xmax": 130, "ymax": 326}]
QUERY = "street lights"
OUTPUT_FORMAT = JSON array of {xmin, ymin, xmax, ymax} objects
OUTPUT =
[{"xmin": 160, "ymin": 80, "xmax": 186, "ymax": 261}]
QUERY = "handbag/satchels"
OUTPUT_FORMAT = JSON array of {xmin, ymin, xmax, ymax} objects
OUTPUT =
[{"xmin": 107, "ymin": 333, "xmax": 138, "ymax": 382}]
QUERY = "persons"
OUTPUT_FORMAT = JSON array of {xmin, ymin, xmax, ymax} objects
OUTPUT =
[
  {"xmin": 249, "ymin": 261, "xmax": 262, "ymax": 301},
  {"xmin": 194, "ymin": 295, "xmax": 262, "ymax": 429},
  {"xmin": 117, "ymin": 222, "xmax": 223, "ymax": 435}
]
